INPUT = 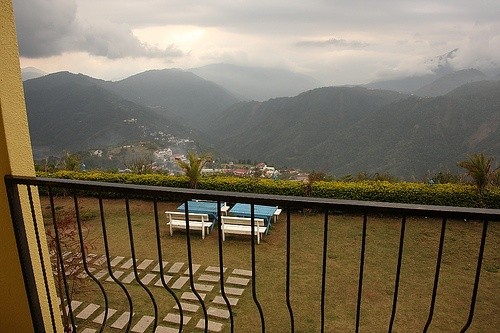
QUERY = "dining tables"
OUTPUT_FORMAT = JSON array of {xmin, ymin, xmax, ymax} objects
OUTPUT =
[
  {"xmin": 177, "ymin": 201, "xmax": 225, "ymax": 234},
  {"xmin": 228, "ymin": 203, "xmax": 278, "ymax": 235}
]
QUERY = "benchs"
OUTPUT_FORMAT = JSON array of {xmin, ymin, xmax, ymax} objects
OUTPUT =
[
  {"xmin": 216, "ymin": 215, "xmax": 267, "ymax": 245},
  {"xmin": 164, "ymin": 211, "xmax": 213, "ymax": 240}
]
